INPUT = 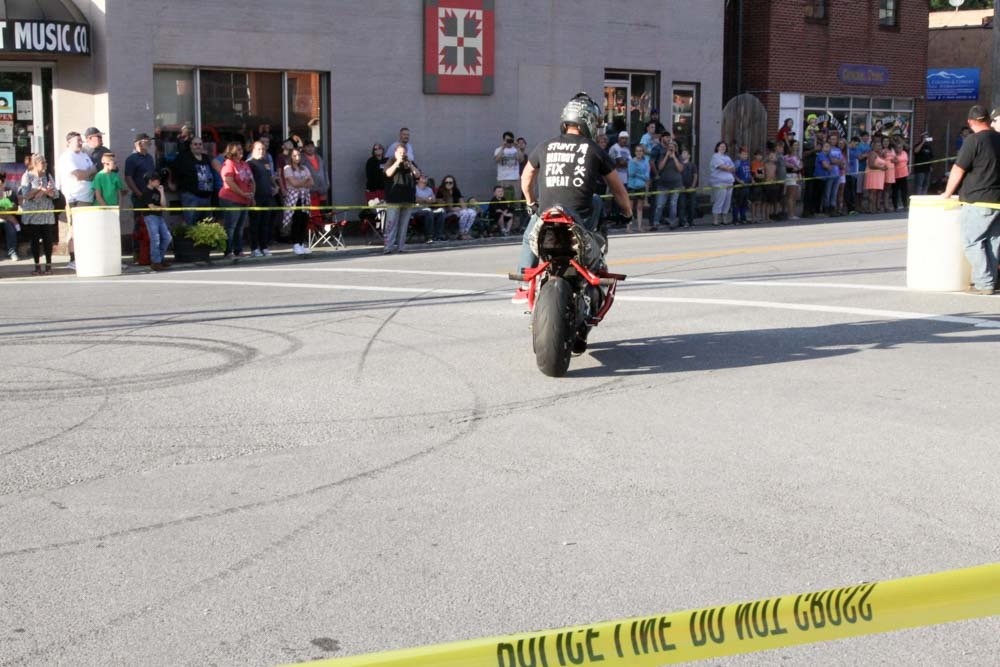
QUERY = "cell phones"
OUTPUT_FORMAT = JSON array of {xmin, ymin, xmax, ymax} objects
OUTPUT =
[{"xmin": 925, "ymin": 136, "xmax": 933, "ymax": 143}]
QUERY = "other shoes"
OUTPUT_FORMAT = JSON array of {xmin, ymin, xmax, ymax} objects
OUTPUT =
[
  {"xmin": 15, "ymin": 224, "xmax": 21, "ymax": 231},
  {"xmin": 511, "ymin": 288, "xmax": 529, "ymax": 305},
  {"xmin": 44, "ymin": 265, "xmax": 52, "ymax": 274},
  {"xmin": 625, "ymin": 203, "xmax": 909, "ymax": 234},
  {"xmin": 148, "ymin": 224, "xmax": 530, "ymax": 272},
  {"xmin": 965, "ymin": 286, "xmax": 995, "ymax": 295},
  {"xmin": 67, "ymin": 260, "xmax": 76, "ymax": 269},
  {"xmin": 797, "ymin": 180, "xmax": 802, "ymax": 185},
  {"xmin": 121, "ymin": 263, "xmax": 128, "ymax": 269},
  {"xmin": 10, "ymin": 252, "xmax": 19, "ymax": 261},
  {"xmin": 32, "ymin": 265, "xmax": 41, "ymax": 275}
]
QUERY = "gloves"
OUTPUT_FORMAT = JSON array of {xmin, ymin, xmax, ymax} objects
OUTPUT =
[
  {"xmin": 615, "ymin": 212, "xmax": 633, "ymax": 227},
  {"xmin": 526, "ymin": 203, "xmax": 539, "ymax": 217}
]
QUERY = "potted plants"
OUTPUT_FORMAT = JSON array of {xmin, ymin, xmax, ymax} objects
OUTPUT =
[{"xmin": 175, "ymin": 216, "xmax": 226, "ymax": 262}]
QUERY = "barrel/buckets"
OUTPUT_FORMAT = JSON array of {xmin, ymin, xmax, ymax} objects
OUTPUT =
[
  {"xmin": 70, "ymin": 206, "xmax": 122, "ymax": 277},
  {"xmin": 907, "ymin": 195, "xmax": 972, "ymax": 293}
]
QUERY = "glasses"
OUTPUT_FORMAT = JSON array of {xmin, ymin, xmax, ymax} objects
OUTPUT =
[
  {"xmin": 374, "ymin": 147, "xmax": 382, "ymax": 151},
  {"xmin": 444, "ymin": 182, "xmax": 454, "ymax": 184}
]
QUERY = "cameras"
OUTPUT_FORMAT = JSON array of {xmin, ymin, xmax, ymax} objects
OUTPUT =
[
  {"xmin": 402, "ymin": 154, "xmax": 409, "ymax": 161},
  {"xmin": 506, "ymin": 138, "xmax": 515, "ymax": 143}
]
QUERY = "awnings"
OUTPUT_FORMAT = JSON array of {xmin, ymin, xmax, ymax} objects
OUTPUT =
[{"xmin": 0, "ymin": 0, "xmax": 91, "ymax": 56}]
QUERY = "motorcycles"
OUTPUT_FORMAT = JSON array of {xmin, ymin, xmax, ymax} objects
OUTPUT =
[{"xmin": 508, "ymin": 200, "xmax": 635, "ymax": 378}]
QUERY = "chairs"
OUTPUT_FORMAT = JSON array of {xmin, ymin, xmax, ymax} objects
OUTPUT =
[
  {"xmin": 365, "ymin": 191, "xmax": 459, "ymax": 243},
  {"xmin": 308, "ymin": 194, "xmax": 350, "ymax": 249}
]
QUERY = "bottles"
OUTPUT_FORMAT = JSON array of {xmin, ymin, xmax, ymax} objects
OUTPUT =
[{"xmin": 47, "ymin": 181, "xmax": 53, "ymax": 198}]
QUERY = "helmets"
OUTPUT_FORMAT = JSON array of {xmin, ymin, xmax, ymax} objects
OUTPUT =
[{"xmin": 559, "ymin": 92, "xmax": 600, "ymax": 142}]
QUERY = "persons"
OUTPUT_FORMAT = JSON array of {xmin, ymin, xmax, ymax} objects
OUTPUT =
[
  {"xmin": 518, "ymin": 91, "xmax": 633, "ymax": 297},
  {"xmin": 595, "ymin": 110, "xmax": 698, "ymax": 234},
  {"xmin": 593, "ymin": 109, "xmax": 646, "ymax": 148},
  {"xmin": 126, "ymin": 122, "xmax": 330, "ymax": 271},
  {"xmin": 361, "ymin": 127, "xmax": 533, "ymax": 245},
  {"xmin": 940, "ymin": 106, "xmax": 1000, "ymax": 295},
  {"xmin": 383, "ymin": 144, "xmax": 420, "ymax": 255},
  {"xmin": 912, "ymin": 130, "xmax": 935, "ymax": 194},
  {"xmin": 64, "ymin": 127, "xmax": 127, "ymax": 268},
  {"xmin": 0, "ymin": 153, "xmax": 60, "ymax": 276},
  {"xmin": 709, "ymin": 114, "xmax": 909, "ymax": 226}
]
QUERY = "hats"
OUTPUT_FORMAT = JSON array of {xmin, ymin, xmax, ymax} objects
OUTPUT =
[
  {"xmin": 85, "ymin": 127, "xmax": 105, "ymax": 137},
  {"xmin": 619, "ymin": 131, "xmax": 629, "ymax": 137},
  {"xmin": 132, "ymin": 133, "xmax": 156, "ymax": 143},
  {"xmin": 807, "ymin": 114, "xmax": 817, "ymax": 124}
]
